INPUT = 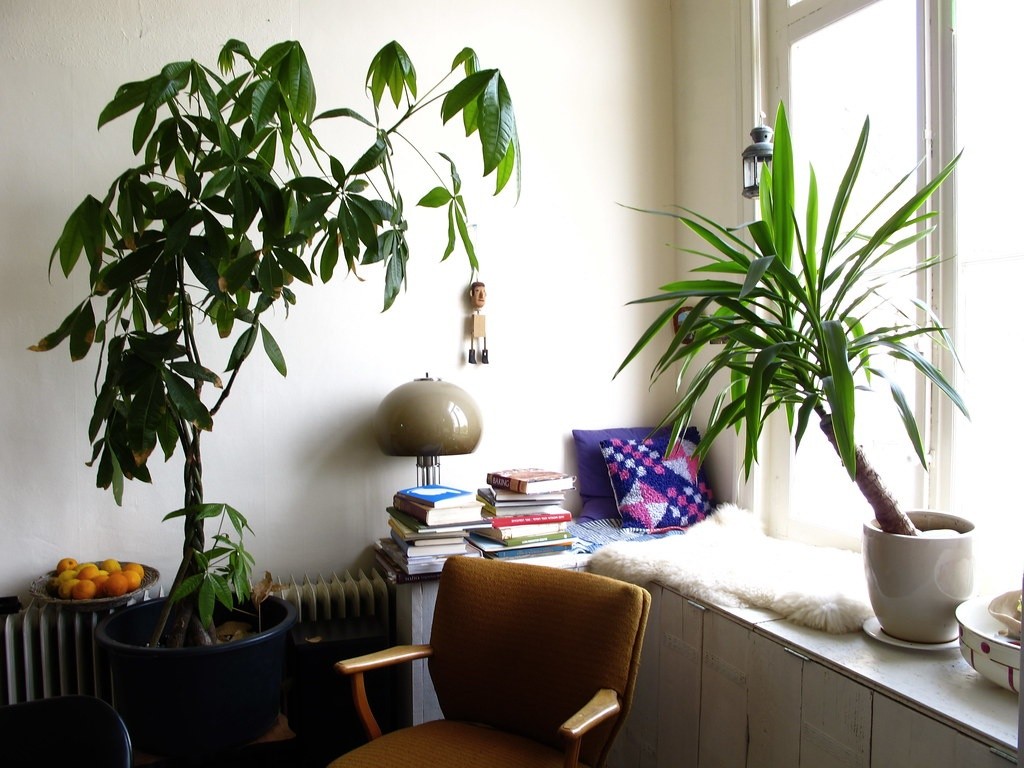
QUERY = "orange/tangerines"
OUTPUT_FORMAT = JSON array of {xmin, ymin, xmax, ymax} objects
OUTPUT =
[{"xmin": 48, "ymin": 558, "xmax": 143, "ymax": 599}]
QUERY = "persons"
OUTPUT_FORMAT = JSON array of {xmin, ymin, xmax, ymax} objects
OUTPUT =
[{"xmin": 469, "ymin": 280, "xmax": 489, "ymax": 365}]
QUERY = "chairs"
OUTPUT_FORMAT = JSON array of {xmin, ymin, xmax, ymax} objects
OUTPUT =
[
  {"xmin": 1, "ymin": 695, "xmax": 133, "ymax": 768},
  {"xmin": 327, "ymin": 555, "xmax": 652, "ymax": 768}
]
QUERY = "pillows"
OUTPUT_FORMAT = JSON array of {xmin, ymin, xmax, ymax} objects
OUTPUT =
[
  {"xmin": 572, "ymin": 428, "xmax": 666, "ymax": 525},
  {"xmin": 600, "ymin": 432, "xmax": 719, "ymax": 531}
]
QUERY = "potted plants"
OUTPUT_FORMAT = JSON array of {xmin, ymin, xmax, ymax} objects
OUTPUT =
[
  {"xmin": 611, "ymin": 98, "xmax": 980, "ymax": 652},
  {"xmin": 27, "ymin": 40, "xmax": 525, "ymax": 750}
]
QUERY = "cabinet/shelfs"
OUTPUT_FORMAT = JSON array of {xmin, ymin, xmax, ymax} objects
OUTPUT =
[{"xmin": 391, "ymin": 557, "xmax": 1021, "ymax": 768}]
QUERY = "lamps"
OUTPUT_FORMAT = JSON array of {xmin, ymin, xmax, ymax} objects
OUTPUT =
[
  {"xmin": 375, "ymin": 373, "xmax": 484, "ymax": 486},
  {"xmin": 740, "ymin": 113, "xmax": 774, "ymax": 201}
]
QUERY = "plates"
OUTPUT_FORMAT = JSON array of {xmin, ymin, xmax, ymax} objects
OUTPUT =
[{"xmin": 862, "ymin": 615, "xmax": 962, "ymax": 650}]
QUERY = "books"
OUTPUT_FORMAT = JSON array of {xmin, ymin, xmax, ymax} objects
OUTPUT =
[{"xmin": 371, "ymin": 468, "xmax": 576, "ymax": 575}]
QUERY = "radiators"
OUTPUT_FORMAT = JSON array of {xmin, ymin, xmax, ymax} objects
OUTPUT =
[{"xmin": 6, "ymin": 569, "xmax": 389, "ymax": 705}]
